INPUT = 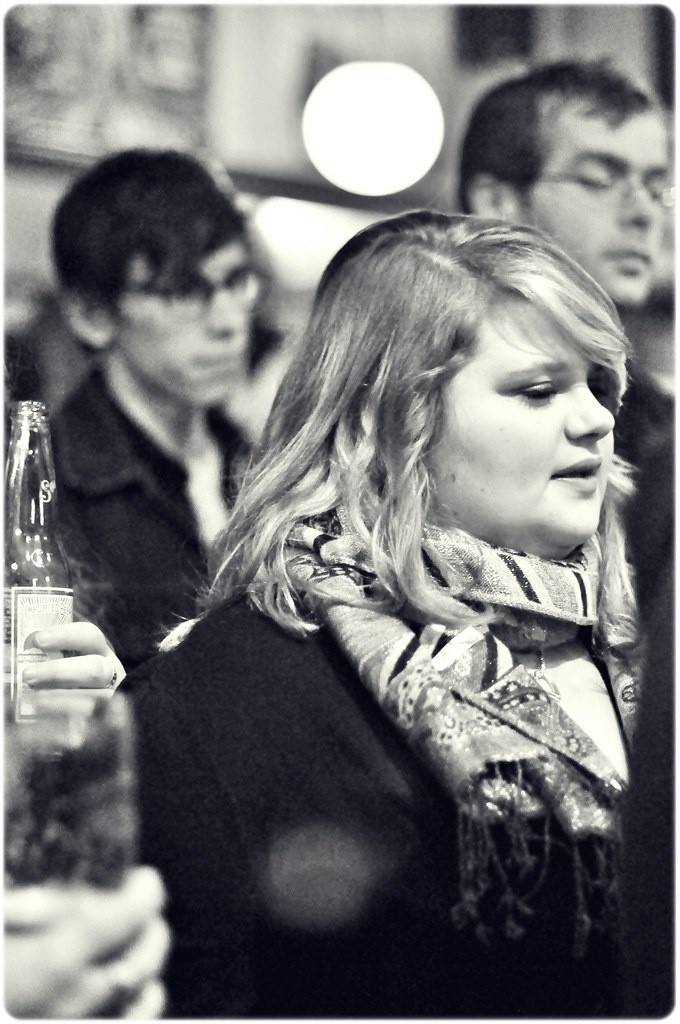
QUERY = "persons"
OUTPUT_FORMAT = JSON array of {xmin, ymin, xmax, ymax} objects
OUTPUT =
[
  {"xmin": 4, "ymin": 622, "xmax": 173, "ymax": 1021},
  {"xmin": 101, "ymin": 208, "xmax": 650, "ymax": 1018},
  {"xmin": 41, "ymin": 144, "xmax": 259, "ymax": 680},
  {"xmin": 463, "ymin": 61, "xmax": 674, "ymax": 614}
]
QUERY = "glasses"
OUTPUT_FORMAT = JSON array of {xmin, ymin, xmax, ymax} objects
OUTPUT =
[
  {"xmin": 536, "ymin": 158, "xmax": 674, "ymax": 208},
  {"xmin": 123, "ymin": 265, "xmax": 271, "ymax": 316}
]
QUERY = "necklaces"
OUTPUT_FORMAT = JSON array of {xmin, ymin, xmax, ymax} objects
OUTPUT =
[{"xmin": 511, "ymin": 647, "xmax": 562, "ymax": 702}]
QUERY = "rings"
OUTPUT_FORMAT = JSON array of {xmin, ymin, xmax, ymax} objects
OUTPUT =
[{"xmin": 109, "ymin": 665, "xmax": 119, "ymax": 686}]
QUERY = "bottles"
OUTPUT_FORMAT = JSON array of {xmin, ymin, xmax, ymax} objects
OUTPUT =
[{"xmin": 4, "ymin": 402, "xmax": 74, "ymax": 746}]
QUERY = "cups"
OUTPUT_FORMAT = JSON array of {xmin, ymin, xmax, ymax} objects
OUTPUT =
[{"xmin": 4, "ymin": 698, "xmax": 135, "ymax": 890}]
